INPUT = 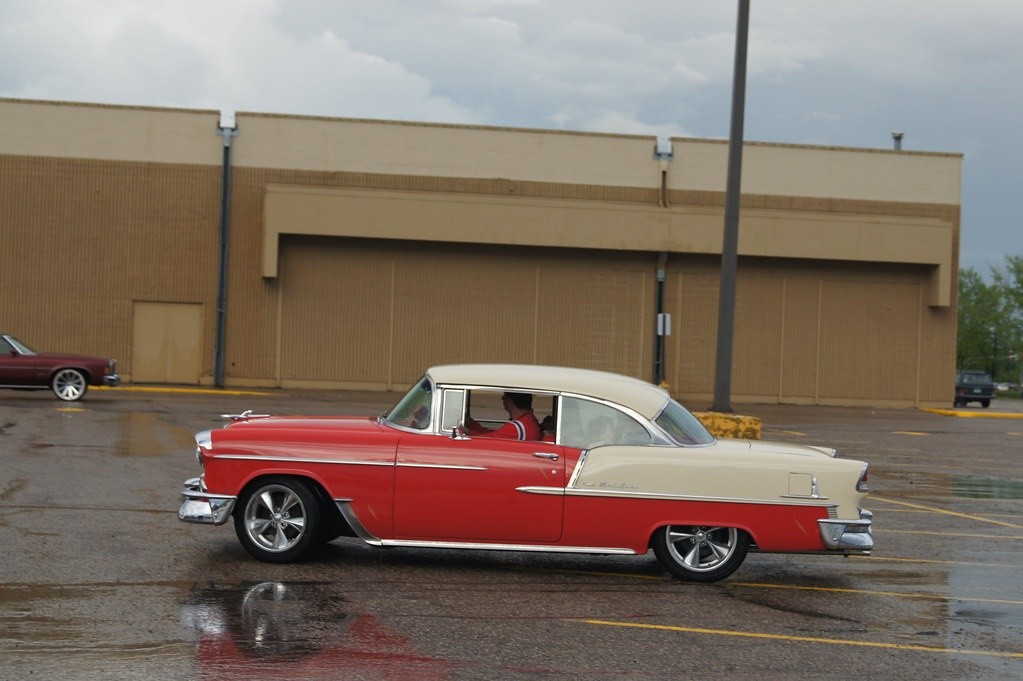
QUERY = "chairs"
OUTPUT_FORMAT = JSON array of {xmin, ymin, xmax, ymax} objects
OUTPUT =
[
  {"xmin": 560, "ymin": 399, "xmax": 585, "ymax": 446},
  {"xmin": 589, "ymin": 416, "xmax": 616, "ymax": 445}
]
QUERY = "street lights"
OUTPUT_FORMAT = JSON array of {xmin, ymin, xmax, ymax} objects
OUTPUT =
[{"xmin": 989, "ymin": 326, "xmax": 998, "ymax": 376}]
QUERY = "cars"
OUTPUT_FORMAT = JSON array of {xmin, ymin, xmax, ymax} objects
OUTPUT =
[
  {"xmin": 0, "ymin": 334, "xmax": 120, "ymax": 402},
  {"xmin": 954, "ymin": 370, "xmax": 996, "ymax": 408},
  {"xmin": 178, "ymin": 363, "xmax": 873, "ymax": 584}
]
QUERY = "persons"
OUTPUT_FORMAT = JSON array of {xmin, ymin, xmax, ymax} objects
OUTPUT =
[{"xmin": 464, "ymin": 392, "xmax": 542, "ymax": 440}]
